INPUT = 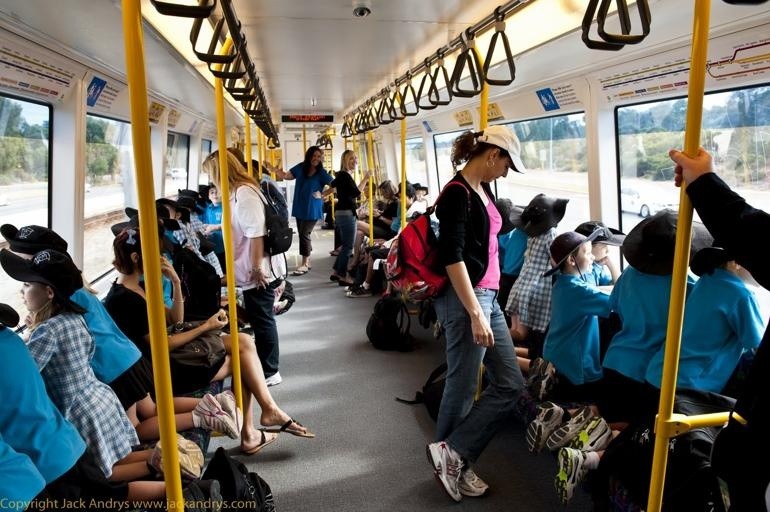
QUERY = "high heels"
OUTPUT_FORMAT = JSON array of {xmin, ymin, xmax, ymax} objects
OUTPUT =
[
  {"xmin": 339, "ymin": 280, "xmax": 358, "ymax": 286},
  {"xmin": 330, "ymin": 274, "xmax": 347, "ymax": 283}
]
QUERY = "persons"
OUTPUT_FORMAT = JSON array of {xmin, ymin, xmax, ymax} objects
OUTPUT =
[
  {"xmin": 554, "ymin": 240, "xmax": 766, "ymax": 512},
  {"xmin": 125, "ymin": 204, "xmax": 181, "ymax": 244},
  {"xmin": 346, "ymin": 184, "xmax": 418, "ymax": 298},
  {"xmin": 505, "ymin": 193, "xmax": 568, "ymax": 342},
  {"xmin": 348, "ymin": 178, "xmax": 400, "ymax": 271},
  {"xmin": 261, "ymin": 146, "xmax": 336, "ymax": 276},
  {"xmin": 495, "ymin": 198, "xmax": 526, "ymax": 311},
  {"xmin": 524, "ymin": 230, "xmax": 618, "ymax": 400},
  {"xmin": 0, "ymin": 248, "xmax": 205, "ymax": 483},
  {"xmin": 203, "ymin": 149, "xmax": 284, "ymax": 387},
  {"xmin": 0, "ymin": 299, "xmax": 224, "ymax": 512},
  {"xmin": 330, "ymin": 149, "xmax": 373, "ymax": 288},
  {"xmin": 99, "ymin": 228, "xmax": 315, "ymax": 456},
  {"xmin": 110, "ymin": 216, "xmax": 174, "ymax": 312},
  {"xmin": 526, "ymin": 210, "xmax": 713, "ymax": 459},
  {"xmin": 515, "ymin": 221, "xmax": 627, "ymax": 375},
  {"xmin": 156, "ymin": 197, "xmax": 247, "ymax": 331},
  {"xmin": 205, "ymin": 181, "xmax": 224, "ymax": 274},
  {"xmin": 330, "ymin": 181, "xmax": 377, "ymax": 258},
  {"xmin": 669, "ymin": 144, "xmax": 770, "ymax": 512},
  {"xmin": 0, "ymin": 224, "xmax": 244, "ymax": 441},
  {"xmin": 423, "ymin": 126, "xmax": 524, "ymax": 503},
  {"xmin": 0, "ymin": 435, "xmax": 49, "ymax": 512}
]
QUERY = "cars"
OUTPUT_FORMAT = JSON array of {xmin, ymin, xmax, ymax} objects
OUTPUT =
[{"xmin": 618, "ymin": 180, "xmax": 682, "ymax": 219}]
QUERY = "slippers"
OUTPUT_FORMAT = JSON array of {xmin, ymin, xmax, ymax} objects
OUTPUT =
[
  {"xmin": 294, "ymin": 266, "xmax": 309, "ymax": 275},
  {"xmin": 245, "ymin": 428, "xmax": 277, "ymax": 454},
  {"xmin": 330, "ymin": 249, "xmax": 353, "ymax": 257},
  {"xmin": 258, "ymin": 417, "xmax": 316, "ymax": 438},
  {"xmin": 296, "ymin": 264, "xmax": 313, "ymax": 269}
]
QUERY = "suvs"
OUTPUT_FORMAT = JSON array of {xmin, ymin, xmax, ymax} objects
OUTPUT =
[{"xmin": 171, "ymin": 168, "xmax": 187, "ymax": 180}]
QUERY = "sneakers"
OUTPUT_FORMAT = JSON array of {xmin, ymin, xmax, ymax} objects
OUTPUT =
[
  {"xmin": 176, "ymin": 432, "xmax": 205, "ymax": 468},
  {"xmin": 147, "ymin": 442, "xmax": 202, "ymax": 480},
  {"xmin": 344, "ymin": 285, "xmax": 360, "ymax": 291},
  {"xmin": 191, "ymin": 479, "xmax": 223, "ymax": 512},
  {"xmin": 174, "ymin": 483, "xmax": 207, "ymax": 512},
  {"xmin": 527, "ymin": 402, "xmax": 564, "ymax": 455},
  {"xmin": 529, "ymin": 362, "xmax": 556, "ymax": 400},
  {"xmin": 346, "ymin": 287, "xmax": 373, "ymax": 297},
  {"xmin": 425, "ymin": 443, "xmax": 464, "ymax": 501},
  {"xmin": 458, "ymin": 468, "xmax": 490, "ymax": 497},
  {"xmin": 569, "ymin": 418, "xmax": 610, "ymax": 450},
  {"xmin": 546, "ymin": 408, "xmax": 596, "ymax": 448},
  {"xmin": 193, "ymin": 392, "xmax": 239, "ymax": 439},
  {"xmin": 525, "ymin": 358, "xmax": 543, "ymax": 382},
  {"xmin": 264, "ymin": 370, "xmax": 282, "ymax": 385},
  {"xmin": 214, "ymin": 389, "xmax": 242, "ymax": 433},
  {"xmin": 552, "ymin": 448, "xmax": 584, "ymax": 501}
]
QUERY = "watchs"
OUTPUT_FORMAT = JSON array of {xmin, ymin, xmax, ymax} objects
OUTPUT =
[{"xmin": 252, "ymin": 265, "xmax": 262, "ymax": 273}]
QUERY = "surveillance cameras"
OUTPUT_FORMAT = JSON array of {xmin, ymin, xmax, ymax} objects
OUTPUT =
[{"xmin": 354, "ymin": 1, "xmax": 371, "ymax": 17}]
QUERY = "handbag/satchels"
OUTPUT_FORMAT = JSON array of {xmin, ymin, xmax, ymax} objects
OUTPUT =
[{"xmin": 156, "ymin": 322, "xmax": 226, "ymax": 372}]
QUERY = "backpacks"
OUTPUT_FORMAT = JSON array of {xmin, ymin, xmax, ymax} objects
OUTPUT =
[
  {"xmin": 234, "ymin": 180, "xmax": 293, "ymax": 256},
  {"xmin": 386, "ymin": 181, "xmax": 472, "ymax": 305}
]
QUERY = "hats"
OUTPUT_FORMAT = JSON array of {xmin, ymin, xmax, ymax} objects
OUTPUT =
[
  {"xmin": 690, "ymin": 240, "xmax": 732, "ymax": 278},
  {"xmin": 0, "ymin": 303, "xmax": 19, "ymax": 328},
  {"xmin": 622, "ymin": 208, "xmax": 716, "ymax": 273},
  {"xmin": 543, "ymin": 228, "xmax": 603, "ymax": 277},
  {"xmin": 125, "ymin": 200, "xmax": 180, "ymax": 230},
  {"xmin": 110, "ymin": 216, "xmax": 165, "ymax": 240},
  {"xmin": 0, "ymin": 222, "xmax": 67, "ymax": 255},
  {"xmin": 394, "ymin": 185, "xmax": 417, "ymax": 197},
  {"xmin": 510, "ymin": 193, "xmax": 569, "ymax": 236},
  {"xmin": 576, "ymin": 222, "xmax": 623, "ymax": 248},
  {"xmin": 0, "ymin": 248, "xmax": 83, "ymax": 297},
  {"xmin": 477, "ymin": 126, "xmax": 526, "ymax": 175},
  {"xmin": 178, "ymin": 188, "xmax": 208, "ymax": 208},
  {"xmin": 412, "ymin": 183, "xmax": 428, "ymax": 197},
  {"xmin": 177, "ymin": 196, "xmax": 204, "ymax": 216},
  {"xmin": 200, "ymin": 184, "xmax": 215, "ymax": 204},
  {"xmin": 157, "ymin": 199, "xmax": 190, "ymax": 223}
]
